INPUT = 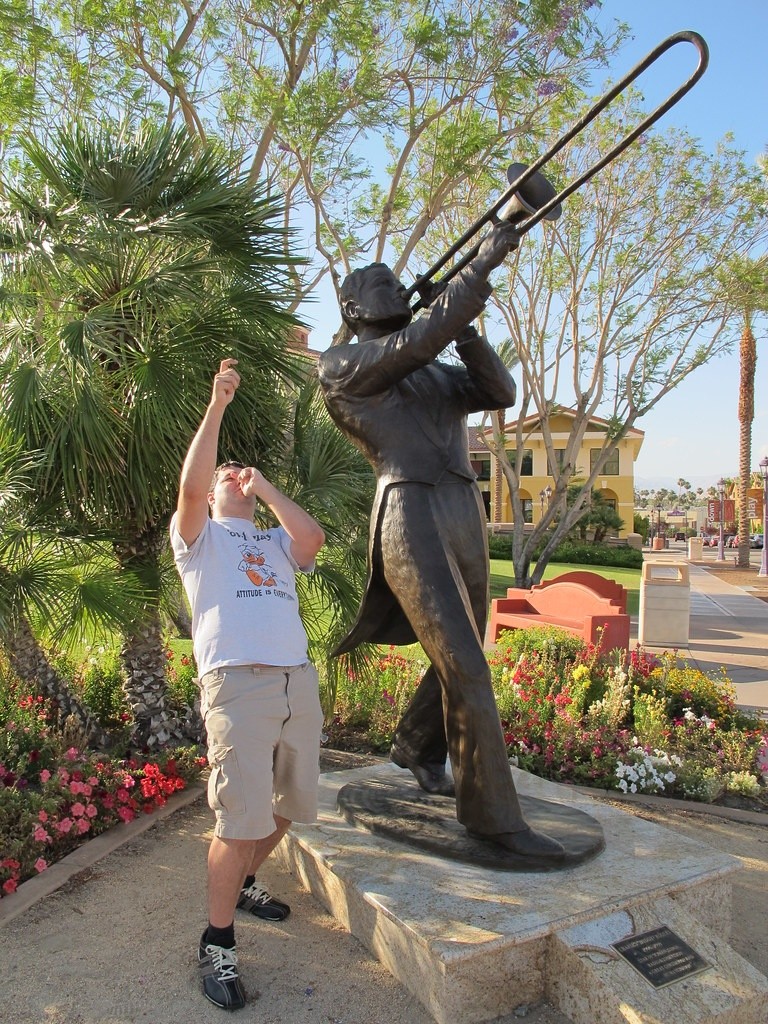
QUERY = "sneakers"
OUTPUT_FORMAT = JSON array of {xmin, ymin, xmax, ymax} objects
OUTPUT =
[
  {"xmin": 236, "ymin": 885, "xmax": 291, "ymax": 921},
  {"xmin": 198, "ymin": 926, "xmax": 246, "ymax": 1010}
]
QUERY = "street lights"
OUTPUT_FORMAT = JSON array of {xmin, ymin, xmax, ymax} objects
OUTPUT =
[
  {"xmin": 715, "ymin": 477, "xmax": 728, "ymax": 560},
  {"xmin": 656, "ymin": 503, "xmax": 662, "ymax": 539},
  {"xmin": 544, "ymin": 484, "xmax": 553, "ymax": 510},
  {"xmin": 759, "ymin": 455, "xmax": 768, "ymax": 577},
  {"xmin": 650, "ymin": 509, "xmax": 654, "ymax": 548},
  {"xmin": 539, "ymin": 489, "xmax": 545, "ymax": 518}
]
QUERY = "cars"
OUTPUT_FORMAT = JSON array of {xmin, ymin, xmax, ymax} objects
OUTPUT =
[
  {"xmin": 753, "ymin": 534, "xmax": 764, "ymax": 549},
  {"xmin": 709, "ymin": 535, "xmax": 720, "ymax": 547},
  {"xmin": 730, "ymin": 535, "xmax": 738, "ymax": 548},
  {"xmin": 725, "ymin": 536, "xmax": 735, "ymax": 548},
  {"xmin": 674, "ymin": 532, "xmax": 687, "ymax": 541},
  {"xmin": 748, "ymin": 535, "xmax": 756, "ymax": 549},
  {"xmin": 702, "ymin": 537, "xmax": 710, "ymax": 546}
]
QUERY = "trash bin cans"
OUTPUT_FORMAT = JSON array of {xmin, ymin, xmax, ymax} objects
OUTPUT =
[
  {"xmin": 688, "ymin": 537, "xmax": 702, "ymax": 561},
  {"xmin": 638, "ymin": 560, "xmax": 691, "ymax": 648}
]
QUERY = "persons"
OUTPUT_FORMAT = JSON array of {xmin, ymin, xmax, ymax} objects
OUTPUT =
[
  {"xmin": 320, "ymin": 217, "xmax": 565, "ymax": 856},
  {"xmin": 168, "ymin": 359, "xmax": 325, "ymax": 1013}
]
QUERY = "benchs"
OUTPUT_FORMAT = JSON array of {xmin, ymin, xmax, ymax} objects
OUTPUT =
[{"xmin": 489, "ymin": 571, "xmax": 631, "ymax": 662}]
